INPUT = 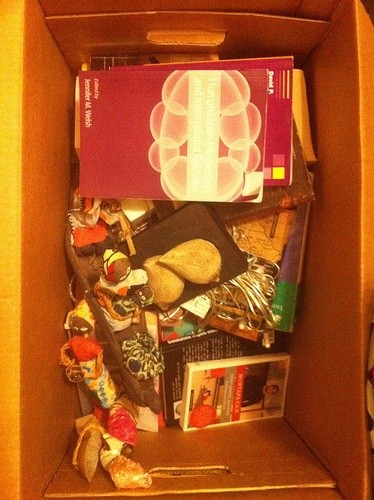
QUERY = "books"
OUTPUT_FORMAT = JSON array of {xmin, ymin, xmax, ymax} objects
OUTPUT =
[{"xmin": 72, "ymin": 55, "xmax": 318, "ymax": 223}]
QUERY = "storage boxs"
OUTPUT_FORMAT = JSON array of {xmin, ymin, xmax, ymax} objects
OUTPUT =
[{"xmin": 1, "ymin": 0, "xmax": 374, "ymax": 500}]
